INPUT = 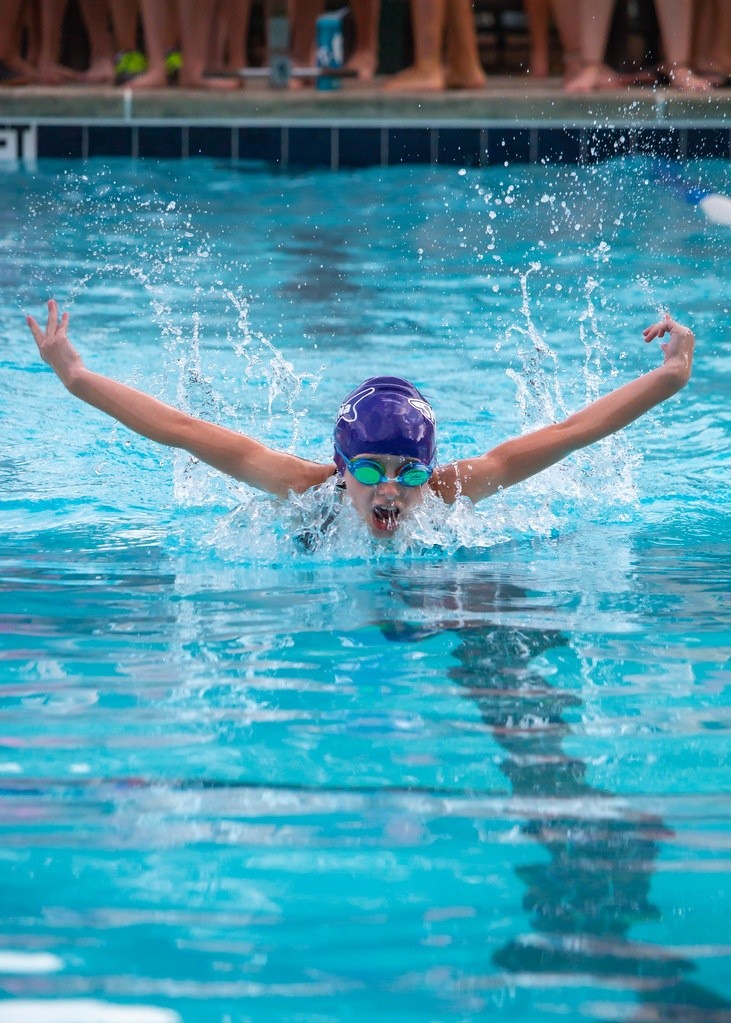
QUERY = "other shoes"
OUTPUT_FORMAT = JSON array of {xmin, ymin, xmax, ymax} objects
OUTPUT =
[
  {"xmin": 114, "ymin": 49, "xmax": 147, "ymax": 84},
  {"xmin": 164, "ymin": 49, "xmax": 181, "ymax": 85}
]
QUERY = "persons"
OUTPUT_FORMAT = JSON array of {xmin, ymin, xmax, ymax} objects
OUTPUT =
[
  {"xmin": 25, "ymin": 300, "xmax": 695, "ymax": 553},
  {"xmin": 0, "ymin": 0, "xmax": 731, "ymax": 90}
]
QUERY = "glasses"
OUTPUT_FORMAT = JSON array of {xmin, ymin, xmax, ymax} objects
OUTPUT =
[{"xmin": 335, "ymin": 444, "xmax": 433, "ymax": 488}]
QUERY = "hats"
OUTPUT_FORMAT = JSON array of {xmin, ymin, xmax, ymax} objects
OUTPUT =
[{"xmin": 332, "ymin": 376, "xmax": 436, "ymax": 467}]
